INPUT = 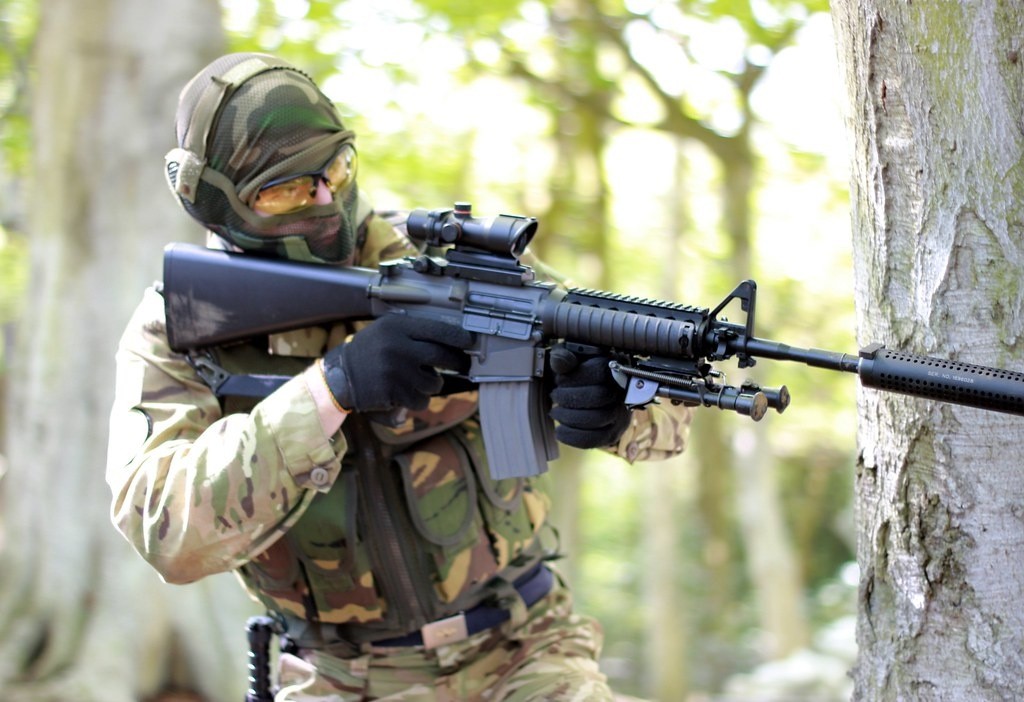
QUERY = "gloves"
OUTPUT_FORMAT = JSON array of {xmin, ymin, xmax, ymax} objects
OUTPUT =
[
  {"xmin": 548, "ymin": 344, "xmax": 628, "ymax": 449},
  {"xmin": 322, "ymin": 314, "xmax": 472, "ymax": 408}
]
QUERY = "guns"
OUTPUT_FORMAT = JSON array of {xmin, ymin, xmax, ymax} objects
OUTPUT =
[{"xmin": 159, "ymin": 200, "xmax": 1024, "ymax": 481}]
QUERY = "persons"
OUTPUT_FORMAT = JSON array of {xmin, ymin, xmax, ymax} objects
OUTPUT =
[{"xmin": 105, "ymin": 52, "xmax": 694, "ymax": 702}]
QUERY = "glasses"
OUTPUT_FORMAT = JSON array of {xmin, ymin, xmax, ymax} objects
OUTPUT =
[{"xmin": 243, "ymin": 142, "xmax": 359, "ymax": 215}]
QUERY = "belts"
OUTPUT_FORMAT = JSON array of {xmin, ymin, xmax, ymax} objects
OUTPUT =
[{"xmin": 369, "ymin": 567, "xmax": 554, "ymax": 650}]
